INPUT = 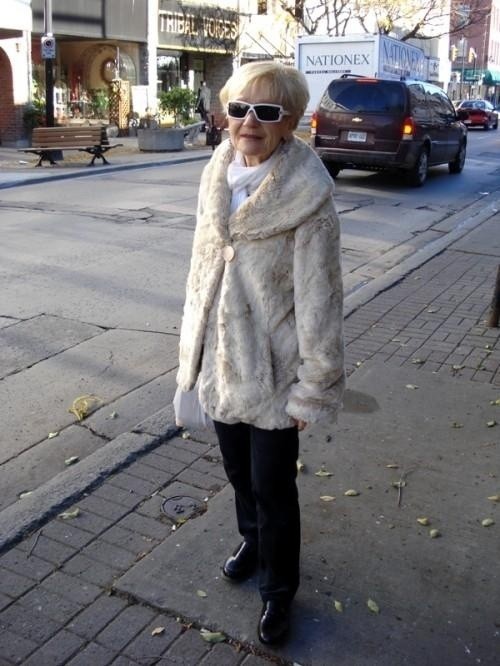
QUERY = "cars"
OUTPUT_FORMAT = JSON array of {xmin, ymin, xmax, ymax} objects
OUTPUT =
[{"xmin": 449, "ymin": 97, "xmax": 499, "ymax": 131}]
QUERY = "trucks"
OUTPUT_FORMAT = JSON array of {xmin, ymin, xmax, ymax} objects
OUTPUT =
[{"xmin": 296, "ymin": 32, "xmax": 430, "ymax": 142}]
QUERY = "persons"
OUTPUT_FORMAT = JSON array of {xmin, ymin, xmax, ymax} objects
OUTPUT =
[{"xmin": 176, "ymin": 59, "xmax": 347, "ymax": 647}]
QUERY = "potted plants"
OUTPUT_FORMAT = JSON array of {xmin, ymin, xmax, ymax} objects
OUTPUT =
[{"xmin": 157, "ymin": 86, "xmax": 209, "ymax": 145}]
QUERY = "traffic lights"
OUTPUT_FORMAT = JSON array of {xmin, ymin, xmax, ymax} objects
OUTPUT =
[
  {"xmin": 455, "ymin": 48, "xmax": 459, "ymax": 60},
  {"xmin": 472, "ymin": 53, "xmax": 474, "ymax": 62}
]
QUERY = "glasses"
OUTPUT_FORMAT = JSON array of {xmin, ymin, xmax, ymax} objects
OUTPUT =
[{"xmin": 223, "ymin": 99, "xmax": 294, "ymax": 125}]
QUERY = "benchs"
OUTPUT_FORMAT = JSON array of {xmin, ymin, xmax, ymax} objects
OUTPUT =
[
  {"xmin": 44, "ymin": 125, "xmax": 123, "ymax": 166},
  {"xmin": 14, "ymin": 122, "xmax": 103, "ymax": 169}
]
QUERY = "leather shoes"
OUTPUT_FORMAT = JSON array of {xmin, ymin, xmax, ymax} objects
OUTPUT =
[
  {"xmin": 221, "ymin": 539, "xmax": 261, "ymax": 583},
  {"xmin": 255, "ymin": 596, "xmax": 293, "ymax": 649}
]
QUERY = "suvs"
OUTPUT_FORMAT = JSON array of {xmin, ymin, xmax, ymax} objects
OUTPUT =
[{"xmin": 314, "ymin": 66, "xmax": 473, "ymax": 181}]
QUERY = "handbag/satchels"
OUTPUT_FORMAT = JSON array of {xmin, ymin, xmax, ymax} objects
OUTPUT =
[{"xmin": 172, "ymin": 374, "xmax": 208, "ymax": 432}]
